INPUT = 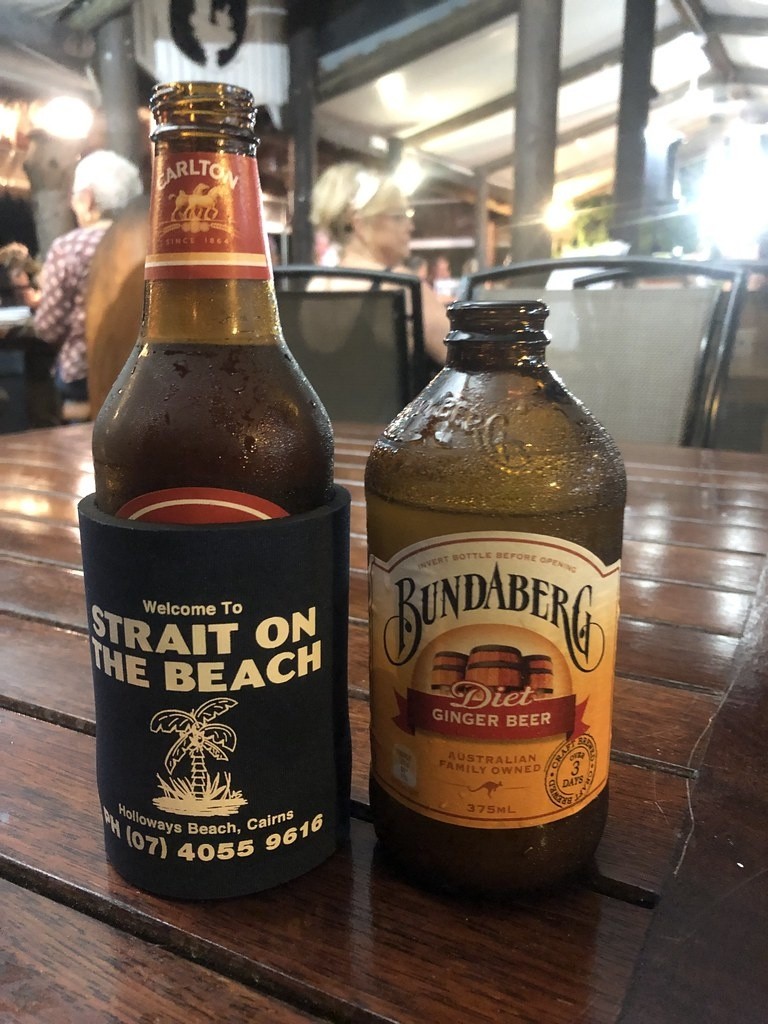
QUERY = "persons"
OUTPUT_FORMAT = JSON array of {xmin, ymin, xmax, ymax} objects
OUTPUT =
[
  {"xmin": 6, "ymin": 150, "xmax": 144, "ymax": 426},
  {"xmin": 304, "ymin": 162, "xmax": 479, "ymax": 405}
]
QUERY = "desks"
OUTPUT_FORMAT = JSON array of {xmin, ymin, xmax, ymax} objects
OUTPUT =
[
  {"xmin": 0, "ymin": 308, "xmax": 69, "ymax": 428},
  {"xmin": 0, "ymin": 416, "xmax": 768, "ymax": 1024}
]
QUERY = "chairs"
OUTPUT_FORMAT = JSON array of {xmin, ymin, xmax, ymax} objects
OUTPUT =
[{"xmin": 269, "ymin": 259, "xmax": 768, "ymax": 454}]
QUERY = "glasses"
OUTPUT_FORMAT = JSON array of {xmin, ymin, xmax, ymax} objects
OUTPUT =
[{"xmin": 385, "ymin": 208, "xmax": 416, "ymax": 221}]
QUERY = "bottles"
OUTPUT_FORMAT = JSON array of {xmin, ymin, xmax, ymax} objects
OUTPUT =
[
  {"xmin": 90, "ymin": 80, "xmax": 337, "ymax": 527},
  {"xmin": 360, "ymin": 300, "xmax": 627, "ymax": 899}
]
